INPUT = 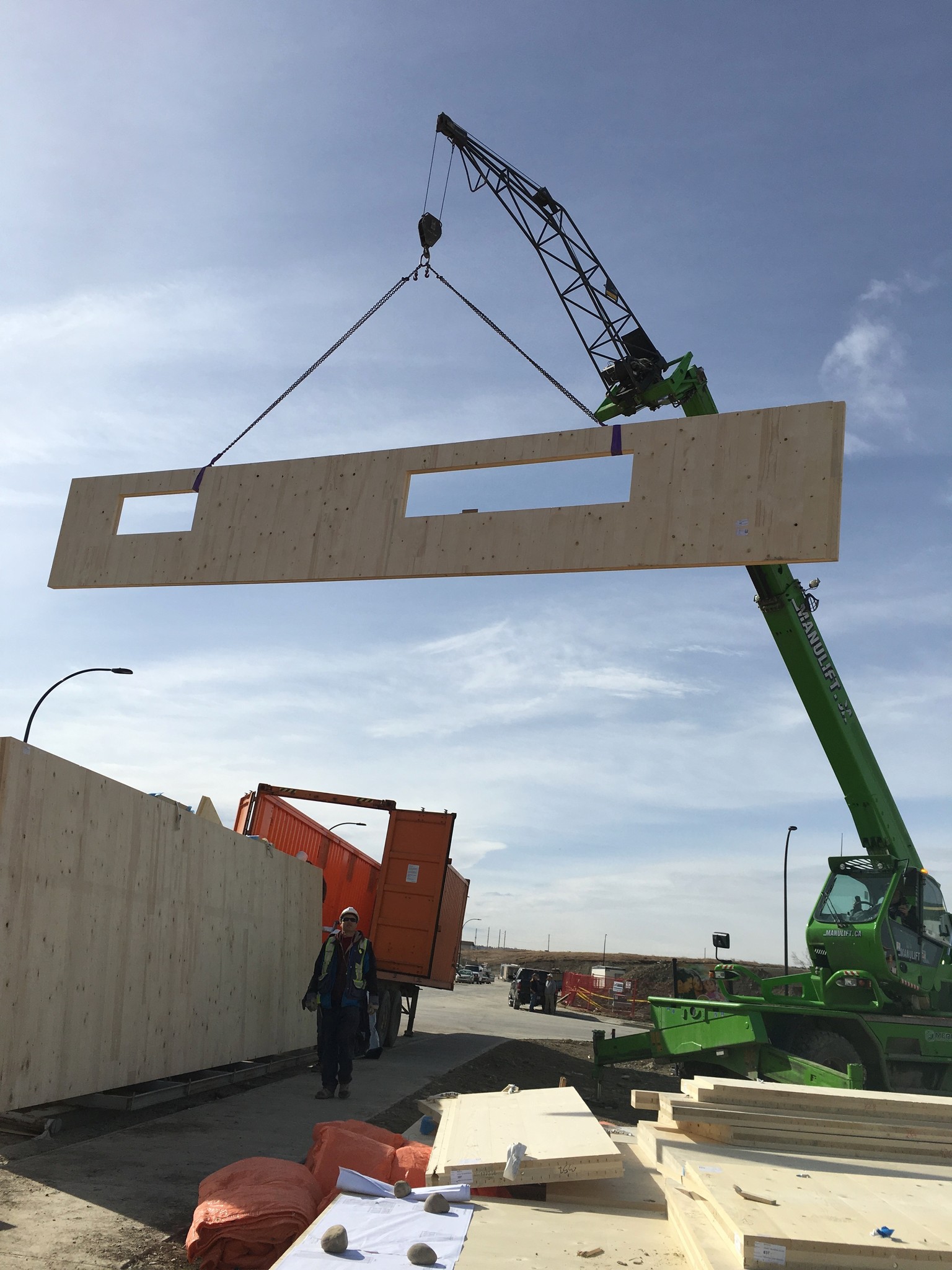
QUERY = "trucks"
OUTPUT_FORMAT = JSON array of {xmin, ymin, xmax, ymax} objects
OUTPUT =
[{"xmin": 234, "ymin": 782, "xmax": 470, "ymax": 1046}]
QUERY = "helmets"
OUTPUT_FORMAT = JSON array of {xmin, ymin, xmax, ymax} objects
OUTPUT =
[
  {"xmin": 339, "ymin": 906, "xmax": 359, "ymax": 922},
  {"xmin": 328, "ymin": 929, "xmax": 341, "ymax": 937}
]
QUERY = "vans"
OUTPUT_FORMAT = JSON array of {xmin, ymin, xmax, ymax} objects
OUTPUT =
[{"xmin": 462, "ymin": 965, "xmax": 483, "ymax": 985}]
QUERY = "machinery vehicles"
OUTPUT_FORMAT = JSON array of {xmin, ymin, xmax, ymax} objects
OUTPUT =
[{"xmin": 431, "ymin": 110, "xmax": 952, "ymax": 1100}]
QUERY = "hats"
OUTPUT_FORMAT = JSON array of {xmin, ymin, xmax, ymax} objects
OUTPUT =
[{"xmin": 547, "ymin": 973, "xmax": 553, "ymax": 978}]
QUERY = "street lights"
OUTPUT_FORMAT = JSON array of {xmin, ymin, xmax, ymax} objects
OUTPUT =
[
  {"xmin": 22, "ymin": 666, "xmax": 135, "ymax": 743},
  {"xmin": 784, "ymin": 826, "xmax": 798, "ymax": 997},
  {"xmin": 603, "ymin": 934, "xmax": 608, "ymax": 965},
  {"xmin": 458, "ymin": 918, "xmax": 482, "ymax": 967}
]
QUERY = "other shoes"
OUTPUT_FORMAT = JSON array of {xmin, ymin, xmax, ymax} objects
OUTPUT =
[{"xmin": 311, "ymin": 1060, "xmax": 324, "ymax": 1073}]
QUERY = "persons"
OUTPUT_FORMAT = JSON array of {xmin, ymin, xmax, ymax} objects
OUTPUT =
[
  {"xmin": 529, "ymin": 973, "xmax": 540, "ymax": 1012},
  {"xmin": 676, "ymin": 967, "xmax": 729, "ymax": 1002},
  {"xmin": 866, "ymin": 884, "xmax": 917, "ymax": 929},
  {"xmin": 543, "ymin": 973, "xmax": 557, "ymax": 1015},
  {"xmin": 486, "ymin": 972, "xmax": 491, "ymax": 984},
  {"xmin": 300, "ymin": 906, "xmax": 381, "ymax": 1101}
]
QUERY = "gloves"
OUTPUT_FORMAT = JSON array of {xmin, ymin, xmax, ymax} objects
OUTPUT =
[
  {"xmin": 367, "ymin": 996, "xmax": 379, "ymax": 1016},
  {"xmin": 301, "ymin": 990, "xmax": 318, "ymax": 1013}
]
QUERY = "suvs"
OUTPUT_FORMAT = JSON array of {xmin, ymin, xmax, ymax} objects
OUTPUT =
[{"xmin": 506, "ymin": 967, "xmax": 559, "ymax": 1013}]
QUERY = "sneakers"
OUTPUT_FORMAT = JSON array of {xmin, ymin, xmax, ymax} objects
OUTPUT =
[
  {"xmin": 339, "ymin": 1079, "xmax": 353, "ymax": 1100},
  {"xmin": 315, "ymin": 1087, "xmax": 336, "ymax": 1099}
]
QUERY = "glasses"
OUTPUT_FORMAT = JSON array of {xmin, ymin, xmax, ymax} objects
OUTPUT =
[{"xmin": 342, "ymin": 918, "xmax": 357, "ymax": 923}]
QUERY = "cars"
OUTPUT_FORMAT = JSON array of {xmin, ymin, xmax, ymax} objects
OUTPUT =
[
  {"xmin": 479, "ymin": 971, "xmax": 495, "ymax": 984},
  {"xmin": 455, "ymin": 969, "xmax": 474, "ymax": 984}
]
